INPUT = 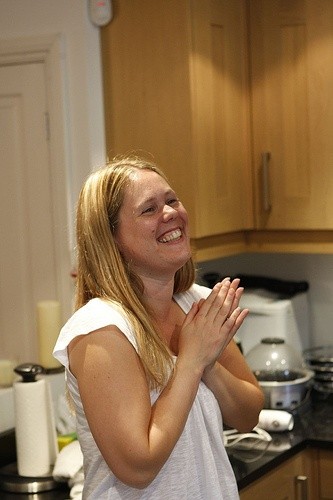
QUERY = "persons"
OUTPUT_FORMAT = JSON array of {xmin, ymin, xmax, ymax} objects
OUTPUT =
[{"xmin": 52, "ymin": 155, "xmax": 265, "ymax": 500}]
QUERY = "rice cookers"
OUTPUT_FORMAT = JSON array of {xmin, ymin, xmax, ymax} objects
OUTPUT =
[{"xmin": 201, "ymin": 271, "xmax": 311, "ymax": 351}]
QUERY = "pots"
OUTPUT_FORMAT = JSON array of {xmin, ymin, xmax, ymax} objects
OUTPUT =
[{"xmin": 242, "ymin": 337, "xmax": 315, "ymax": 412}]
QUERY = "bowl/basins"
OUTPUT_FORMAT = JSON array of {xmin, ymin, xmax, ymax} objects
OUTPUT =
[{"xmin": 301, "ymin": 345, "xmax": 333, "ymax": 396}]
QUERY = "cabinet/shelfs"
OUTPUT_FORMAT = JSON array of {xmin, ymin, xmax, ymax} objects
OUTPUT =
[
  {"xmin": 247, "ymin": 0, "xmax": 333, "ymax": 254},
  {"xmin": 239, "ymin": 445, "xmax": 317, "ymax": 500},
  {"xmin": 99, "ymin": 0, "xmax": 247, "ymax": 263}
]
